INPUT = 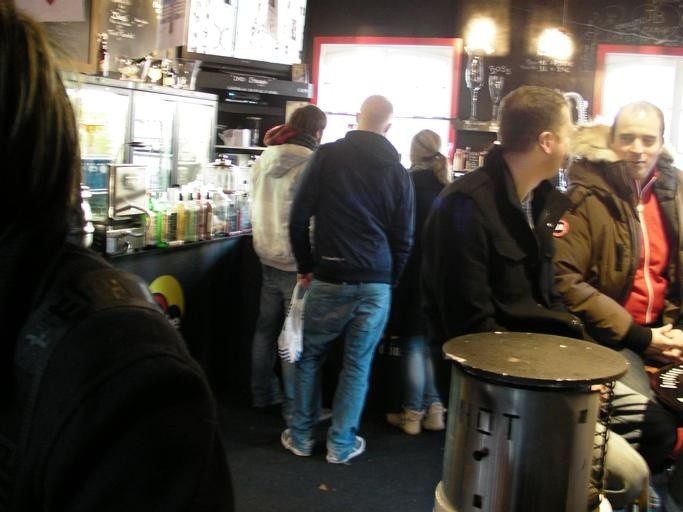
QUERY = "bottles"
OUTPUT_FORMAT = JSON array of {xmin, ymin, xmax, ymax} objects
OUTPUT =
[
  {"xmin": 203, "ymin": 153, "xmax": 259, "ymax": 195},
  {"xmin": 96, "ymin": 37, "xmax": 109, "ymax": 77},
  {"xmin": 146, "ymin": 189, "xmax": 252, "ymax": 247},
  {"xmin": 452, "ymin": 144, "xmax": 487, "ymax": 172}
]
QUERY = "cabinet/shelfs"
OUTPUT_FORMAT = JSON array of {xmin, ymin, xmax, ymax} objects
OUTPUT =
[{"xmin": 452, "ymin": 123, "xmax": 499, "ymax": 177}]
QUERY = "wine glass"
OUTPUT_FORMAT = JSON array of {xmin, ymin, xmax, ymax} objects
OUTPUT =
[
  {"xmin": 464, "ymin": 49, "xmax": 486, "ymax": 123},
  {"xmin": 488, "ymin": 75, "xmax": 505, "ymax": 126}
]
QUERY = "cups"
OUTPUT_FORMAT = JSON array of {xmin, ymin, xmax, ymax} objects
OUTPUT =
[
  {"xmin": 217, "ymin": 117, "xmax": 263, "ymax": 148},
  {"xmin": 116, "ymin": 51, "xmax": 203, "ymax": 90}
]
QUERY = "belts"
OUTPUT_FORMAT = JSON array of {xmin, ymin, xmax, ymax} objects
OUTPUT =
[{"xmin": 313, "ymin": 272, "xmax": 368, "ymax": 286}]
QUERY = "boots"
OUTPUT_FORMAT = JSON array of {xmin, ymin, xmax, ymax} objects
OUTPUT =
[
  {"xmin": 384, "ymin": 403, "xmax": 425, "ymax": 436},
  {"xmin": 421, "ymin": 401, "xmax": 447, "ymax": 432}
]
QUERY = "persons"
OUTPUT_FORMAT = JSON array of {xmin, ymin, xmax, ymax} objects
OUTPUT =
[
  {"xmin": 0, "ymin": 0, "xmax": 235, "ymax": 511},
  {"xmin": 385, "ymin": 129, "xmax": 452, "ymax": 436},
  {"xmin": 248, "ymin": 105, "xmax": 335, "ymax": 420},
  {"xmin": 279, "ymin": 94, "xmax": 414, "ymax": 464},
  {"xmin": 547, "ymin": 97, "xmax": 682, "ymax": 511},
  {"xmin": 419, "ymin": 86, "xmax": 652, "ymax": 511}
]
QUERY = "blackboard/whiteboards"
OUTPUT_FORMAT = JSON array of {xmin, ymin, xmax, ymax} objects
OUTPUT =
[{"xmin": 17, "ymin": 0, "xmax": 100, "ymax": 74}]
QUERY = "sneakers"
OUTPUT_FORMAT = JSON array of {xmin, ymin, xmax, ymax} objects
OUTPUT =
[
  {"xmin": 279, "ymin": 425, "xmax": 316, "ymax": 458},
  {"xmin": 323, "ymin": 434, "xmax": 368, "ymax": 464},
  {"xmin": 647, "ymin": 468, "xmax": 671, "ymax": 510},
  {"xmin": 318, "ymin": 406, "xmax": 332, "ymax": 422}
]
